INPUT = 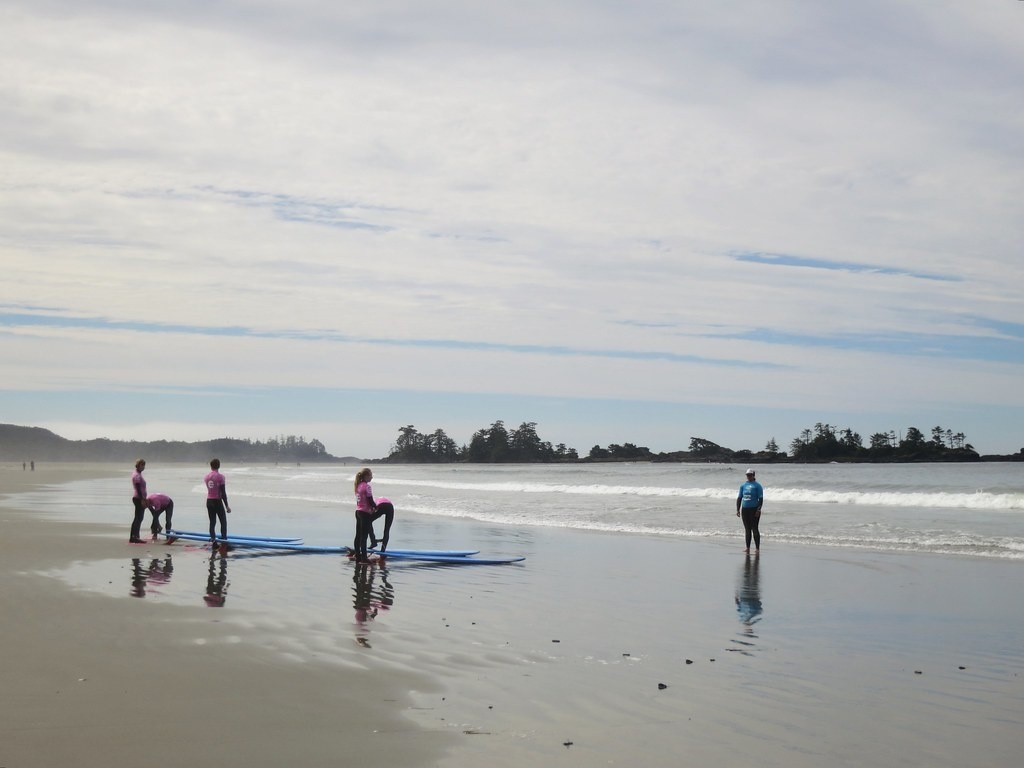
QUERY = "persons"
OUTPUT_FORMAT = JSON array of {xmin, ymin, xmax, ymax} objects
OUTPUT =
[
  {"xmin": 129, "ymin": 458, "xmax": 147, "ymax": 544},
  {"xmin": 368, "ymin": 497, "xmax": 394, "ymax": 552},
  {"xmin": 23, "ymin": 463, "xmax": 26, "ymax": 470},
  {"xmin": 31, "ymin": 461, "xmax": 34, "ymax": 471},
  {"xmin": 146, "ymin": 493, "xmax": 173, "ymax": 541},
  {"xmin": 354, "ymin": 468, "xmax": 379, "ymax": 550},
  {"xmin": 204, "ymin": 459, "xmax": 231, "ymax": 550},
  {"xmin": 736, "ymin": 469, "xmax": 763, "ymax": 552}
]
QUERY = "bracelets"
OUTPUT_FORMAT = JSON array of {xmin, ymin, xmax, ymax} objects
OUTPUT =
[{"xmin": 758, "ymin": 507, "xmax": 761, "ymax": 510}]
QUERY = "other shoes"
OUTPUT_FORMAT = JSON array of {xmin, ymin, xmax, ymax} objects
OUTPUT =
[
  {"xmin": 356, "ymin": 557, "xmax": 362, "ymax": 565},
  {"xmin": 359, "ymin": 558, "xmax": 373, "ymax": 564},
  {"xmin": 129, "ymin": 538, "xmax": 147, "ymax": 543},
  {"xmin": 152, "ymin": 533, "xmax": 157, "ymax": 539},
  {"xmin": 212, "ymin": 541, "xmax": 219, "ymax": 548},
  {"xmin": 368, "ymin": 542, "xmax": 377, "ymax": 549}
]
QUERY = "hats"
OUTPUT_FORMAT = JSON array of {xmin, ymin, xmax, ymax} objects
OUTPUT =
[{"xmin": 745, "ymin": 468, "xmax": 755, "ymax": 475}]
